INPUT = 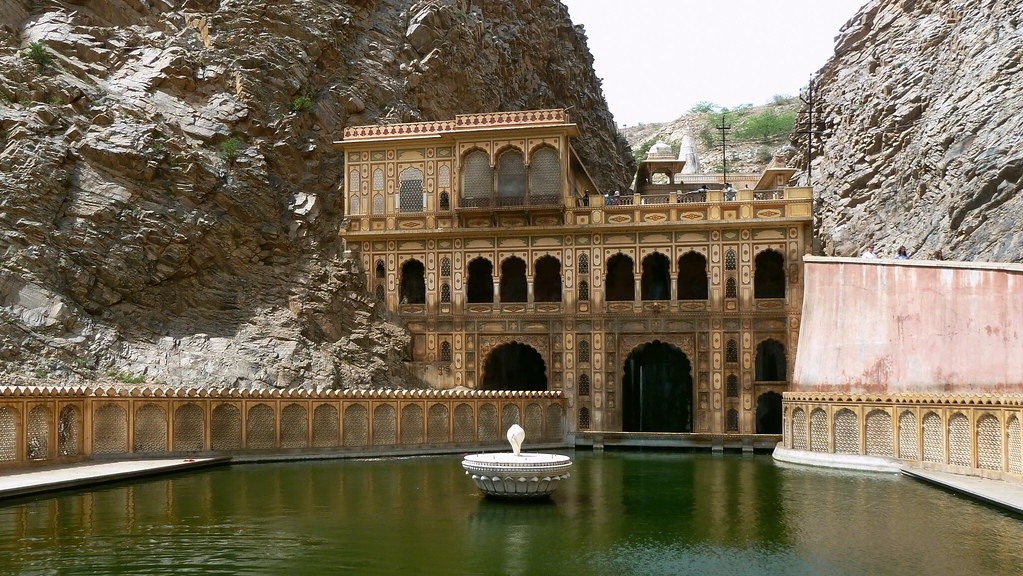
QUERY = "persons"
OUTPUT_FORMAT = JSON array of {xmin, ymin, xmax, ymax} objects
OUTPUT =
[
  {"xmin": 744, "ymin": 184, "xmax": 750, "ymax": 190},
  {"xmin": 604, "ymin": 190, "xmax": 621, "ymax": 205},
  {"xmin": 581, "ymin": 189, "xmax": 589, "ymax": 206},
  {"xmin": 722, "ymin": 183, "xmax": 733, "ymax": 201},
  {"xmin": 699, "ymin": 184, "xmax": 709, "ymax": 202}
]
